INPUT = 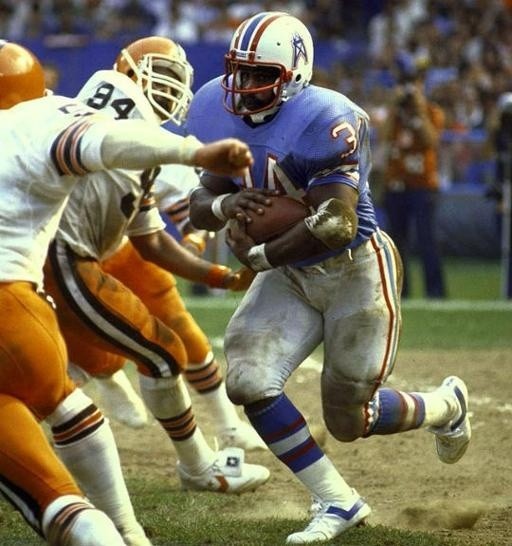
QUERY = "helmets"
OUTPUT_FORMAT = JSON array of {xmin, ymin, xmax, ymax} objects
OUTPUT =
[
  {"xmin": 75, "ymin": 70, "xmax": 155, "ymax": 121},
  {"xmin": 114, "ymin": 36, "xmax": 194, "ymax": 127},
  {"xmin": 220, "ymin": 11, "xmax": 315, "ymax": 116},
  {"xmin": 1, "ymin": 43, "xmax": 49, "ymax": 109}
]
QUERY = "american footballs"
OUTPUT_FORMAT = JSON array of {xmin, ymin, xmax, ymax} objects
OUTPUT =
[{"xmin": 229, "ymin": 194, "xmax": 313, "ymax": 245}]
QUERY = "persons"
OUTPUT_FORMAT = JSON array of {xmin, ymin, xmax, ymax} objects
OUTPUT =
[
  {"xmin": 183, "ymin": 10, "xmax": 473, "ymax": 544},
  {"xmin": 42, "ymin": 34, "xmax": 270, "ymax": 510},
  {"xmin": 0, "ymin": 38, "xmax": 257, "ymax": 546},
  {"xmin": 66, "ymin": 163, "xmax": 270, "ymax": 450}
]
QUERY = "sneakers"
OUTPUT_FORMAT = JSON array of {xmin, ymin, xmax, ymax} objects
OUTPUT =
[
  {"xmin": 434, "ymin": 375, "xmax": 471, "ymax": 465},
  {"xmin": 99, "ymin": 393, "xmax": 150, "ymax": 430},
  {"xmin": 286, "ymin": 488, "xmax": 371, "ymax": 544},
  {"xmin": 174, "ymin": 423, "xmax": 271, "ymax": 494}
]
